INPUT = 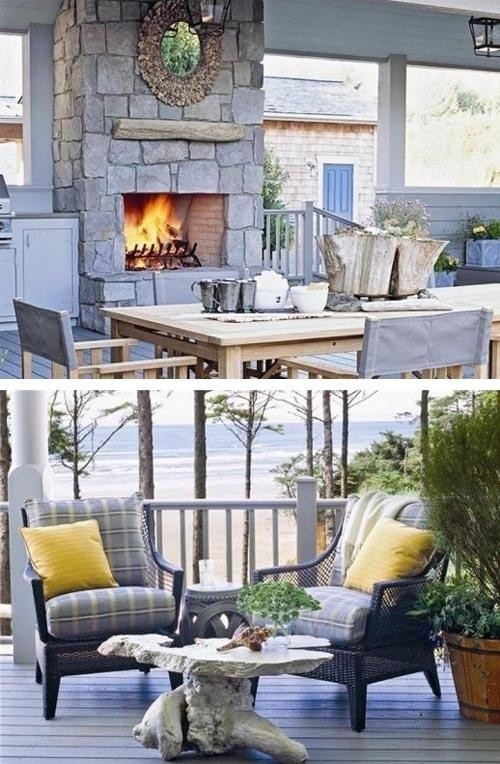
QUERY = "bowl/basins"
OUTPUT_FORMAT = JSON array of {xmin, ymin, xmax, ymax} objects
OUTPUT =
[
  {"xmin": 291, "ymin": 285, "xmax": 329, "ymax": 313},
  {"xmin": 255, "ymin": 286, "xmax": 288, "ymax": 310}
]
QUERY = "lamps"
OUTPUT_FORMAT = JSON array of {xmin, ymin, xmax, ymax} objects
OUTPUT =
[
  {"xmin": 183, "ymin": 0, "xmax": 233, "ymax": 38},
  {"xmin": 467, "ymin": 13, "xmax": 499, "ymax": 60}
]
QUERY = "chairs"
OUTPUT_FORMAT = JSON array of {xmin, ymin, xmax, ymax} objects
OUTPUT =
[
  {"xmin": 241, "ymin": 493, "xmax": 463, "ymax": 738},
  {"xmin": 10, "ymin": 293, "xmax": 201, "ymax": 378},
  {"xmin": 18, "ymin": 489, "xmax": 186, "ymax": 724},
  {"xmin": 277, "ymin": 302, "xmax": 498, "ymax": 378},
  {"xmin": 149, "ymin": 265, "xmax": 284, "ymax": 379}
]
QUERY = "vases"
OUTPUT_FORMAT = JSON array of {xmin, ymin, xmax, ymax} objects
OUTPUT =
[
  {"xmin": 462, "ymin": 238, "xmax": 500, "ymax": 271},
  {"xmin": 433, "ymin": 267, "xmax": 458, "ymax": 287}
]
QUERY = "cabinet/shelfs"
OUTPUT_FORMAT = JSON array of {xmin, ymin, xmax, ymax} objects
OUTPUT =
[{"xmin": 0, "ymin": 208, "xmax": 85, "ymax": 334}]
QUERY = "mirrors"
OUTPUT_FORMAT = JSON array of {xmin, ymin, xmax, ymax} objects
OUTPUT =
[{"xmin": 135, "ymin": 0, "xmax": 224, "ymax": 107}]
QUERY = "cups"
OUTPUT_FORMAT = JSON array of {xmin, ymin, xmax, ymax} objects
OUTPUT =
[
  {"xmin": 191, "ymin": 267, "xmax": 257, "ymax": 313},
  {"xmin": 205, "ymin": 571, "xmax": 217, "ymax": 589},
  {"xmin": 198, "ymin": 558, "xmax": 214, "ymax": 587}
]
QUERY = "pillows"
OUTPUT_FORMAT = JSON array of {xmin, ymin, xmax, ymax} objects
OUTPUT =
[
  {"xmin": 339, "ymin": 512, "xmax": 444, "ymax": 610},
  {"xmin": 20, "ymin": 513, "xmax": 120, "ymax": 603}
]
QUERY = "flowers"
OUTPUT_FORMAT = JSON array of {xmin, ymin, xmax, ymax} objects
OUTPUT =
[
  {"xmin": 360, "ymin": 195, "xmax": 433, "ymax": 239},
  {"xmin": 432, "ymin": 249, "xmax": 461, "ymax": 272},
  {"xmin": 460, "ymin": 207, "xmax": 500, "ymax": 238}
]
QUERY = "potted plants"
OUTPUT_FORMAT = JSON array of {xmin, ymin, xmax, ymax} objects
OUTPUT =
[
  {"xmin": 238, "ymin": 578, "xmax": 328, "ymax": 647},
  {"xmin": 402, "ymin": 389, "xmax": 499, "ymax": 728}
]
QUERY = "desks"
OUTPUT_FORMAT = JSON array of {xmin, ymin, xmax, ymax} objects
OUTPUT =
[
  {"xmin": 175, "ymin": 578, "xmax": 252, "ymax": 651},
  {"xmin": 92, "ymin": 628, "xmax": 339, "ymax": 764},
  {"xmin": 95, "ymin": 285, "xmax": 500, "ymax": 379}
]
